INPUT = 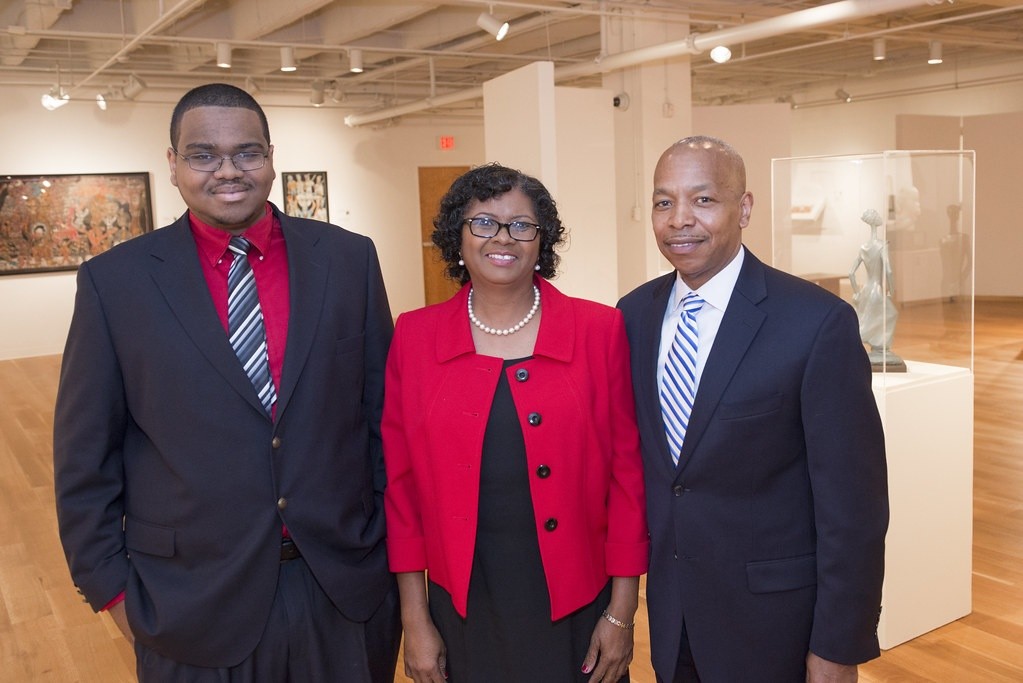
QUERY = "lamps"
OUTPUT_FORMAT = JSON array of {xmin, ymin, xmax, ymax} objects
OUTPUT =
[
  {"xmin": 216, "ymin": 42, "xmax": 232, "ymax": 68},
  {"xmin": 311, "ymin": 81, "xmax": 324, "ymax": 105},
  {"xmin": 280, "ymin": 47, "xmax": 299, "ymax": 71},
  {"xmin": 97, "ymin": 92, "xmax": 119, "ymax": 112},
  {"xmin": 477, "ymin": 11, "xmax": 509, "ymax": 41},
  {"xmin": 836, "ymin": 89, "xmax": 851, "ymax": 102},
  {"xmin": 334, "ymin": 88, "xmax": 343, "ymax": 102},
  {"xmin": 244, "ymin": 77, "xmax": 259, "ymax": 96},
  {"xmin": 927, "ymin": 42, "xmax": 943, "ymax": 64},
  {"xmin": 873, "ymin": 38, "xmax": 886, "ymax": 60},
  {"xmin": 122, "ymin": 78, "xmax": 147, "ymax": 101},
  {"xmin": 349, "ymin": 50, "xmax": 363, "ymax": 72}
]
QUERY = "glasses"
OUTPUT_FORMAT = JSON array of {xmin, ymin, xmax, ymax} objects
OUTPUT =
[
  {"xmin": 462, "ymin": 217, "xmax": 541, "ymax": 241},
  {"xmin": 173, "ymin": 148, "xmax": 269, "ymax": 172}
]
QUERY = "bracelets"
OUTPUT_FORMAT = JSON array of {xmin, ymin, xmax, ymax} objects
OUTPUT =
[{"xmin": 601, "ymin": 610, "xmax": 635, "ymax": 630}]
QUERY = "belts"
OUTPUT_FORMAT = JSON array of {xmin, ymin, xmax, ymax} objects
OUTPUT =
[{"xmin": 280, "ymin": 537, "xmax": 301, "ymax": 559}]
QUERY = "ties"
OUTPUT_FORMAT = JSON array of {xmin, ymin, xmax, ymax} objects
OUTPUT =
[
  {"xmin": 228, "ymin": 235, "xmax": 278, "ymax": 423},
  {"xmin": 659, "ymin": 296, "xmax": 706, "ymax": 470}
]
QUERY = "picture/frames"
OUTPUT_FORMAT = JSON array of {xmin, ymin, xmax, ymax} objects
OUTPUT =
[
  {"xmin": 282, "ymin": 171, "xmax": 329, "ymax": 223},
  {"xmin": 0, "ymin": 172, "xmax": 153, "ymax": 275}
]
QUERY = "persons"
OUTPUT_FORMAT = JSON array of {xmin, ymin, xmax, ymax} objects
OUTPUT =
[
  {"xmin": 379, "ymin": 163, "xmax": 649, "ymax": 683},
  {"xmin": 850, "ymin": 209, "xmax": 898, "ymax": 353},
  {"xmin": 615, "ymin": 135, "xmax": 891, "ymax": 683},
  {"xmin": 52, "ymin": 83, "xmax": 403, "ymax": 683}
]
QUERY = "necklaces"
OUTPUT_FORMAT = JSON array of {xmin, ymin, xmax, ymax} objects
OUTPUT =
[{"xmin": 467, "ymin": 282, "xmax": 540, "ymax": 336}]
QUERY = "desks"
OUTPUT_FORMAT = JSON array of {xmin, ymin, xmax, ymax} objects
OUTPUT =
[{"xmin": 794, "ymin": 274, "xmax": 850, "ymax": 296}]
